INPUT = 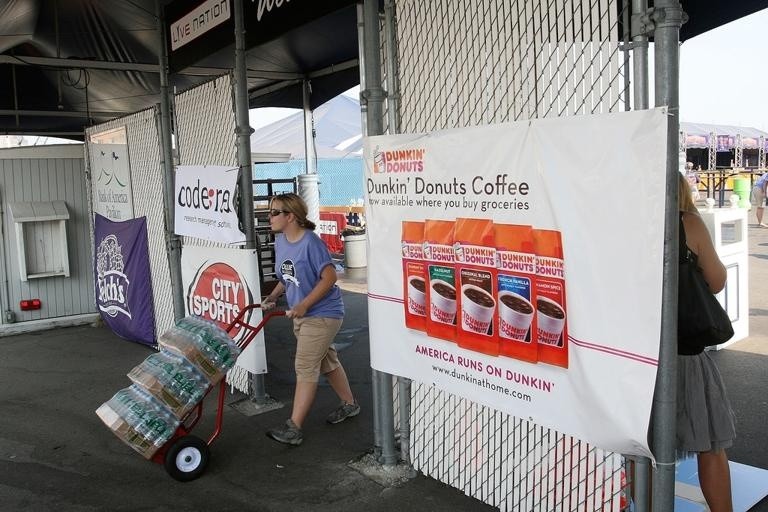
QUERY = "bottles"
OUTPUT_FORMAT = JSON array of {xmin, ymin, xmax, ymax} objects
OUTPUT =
[{"xmin": 107, "ymin": 313, "xmax": 242, "ymax": 450}]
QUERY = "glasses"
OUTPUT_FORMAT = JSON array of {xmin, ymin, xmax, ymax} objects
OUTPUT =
[{"xmin": 271, "ymin": 209, "xmax": 288, "ymax": 216}]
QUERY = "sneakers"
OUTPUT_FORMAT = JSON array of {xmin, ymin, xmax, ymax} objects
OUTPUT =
[
  {"xmin": 266, "ymin": 419, "xmax": 302, "ymax": 445},
  {"xmin": 328, "ymin": 400, "xmax": 360, "ymax": 424}
]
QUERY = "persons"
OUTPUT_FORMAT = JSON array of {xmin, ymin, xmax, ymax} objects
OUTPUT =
[
  {"xmin": 260, "ymin": 192, "xmax": 361, "ymax": 446},
  {"xmin": 684, "ymin": 162, "xmax": 701, "ymax": 203},
  {"xmin": 677, "ymin": 169, "xmax": 737, "ymax": 512},
  {"xmin": 752, "ymin": 172, "xmax": 768, "ymax": 227}
]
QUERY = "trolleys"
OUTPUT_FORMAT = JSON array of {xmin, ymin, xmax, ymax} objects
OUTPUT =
[{"xmin": 152, "ymin": 302, "xmax": 295, "ymax": 485}]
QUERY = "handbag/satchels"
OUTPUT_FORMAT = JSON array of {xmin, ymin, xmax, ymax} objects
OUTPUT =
[{"xmin": 680, "ymin": 263, "xmax": 733, "ymax": 345}]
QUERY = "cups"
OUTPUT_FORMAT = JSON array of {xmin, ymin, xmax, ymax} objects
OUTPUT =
[
  {"xmin": 537, "ymin": 294, "xmax": 566, "ymax": 346},
  {"xmin": 424, "ymin": 247, "xmax": 431, "ymax": 259},
  {"xmin": 430, "ymin": 278, "xmax": 457, "ymax": 324},
  {"xmin": 456, "ymin": 248, "xmax": 464, "ymax": 262},
  {"xmin": 498, "ymin": 289, "xmax": 535, "ymax": 343},
  {"xmin": 402, "ymin": 246, "xmax": 409, "ymax": 257},
  {"xmin": 374, "ymin": 153, "xmax": 385, "ymax": 173},
  {"xmin": 461, "ymin": 283, "xmax": 497, "ymax": 335},
  {"xmin": 407, "ymin": 275, "xmax": 426, "ymax": 317}
]
